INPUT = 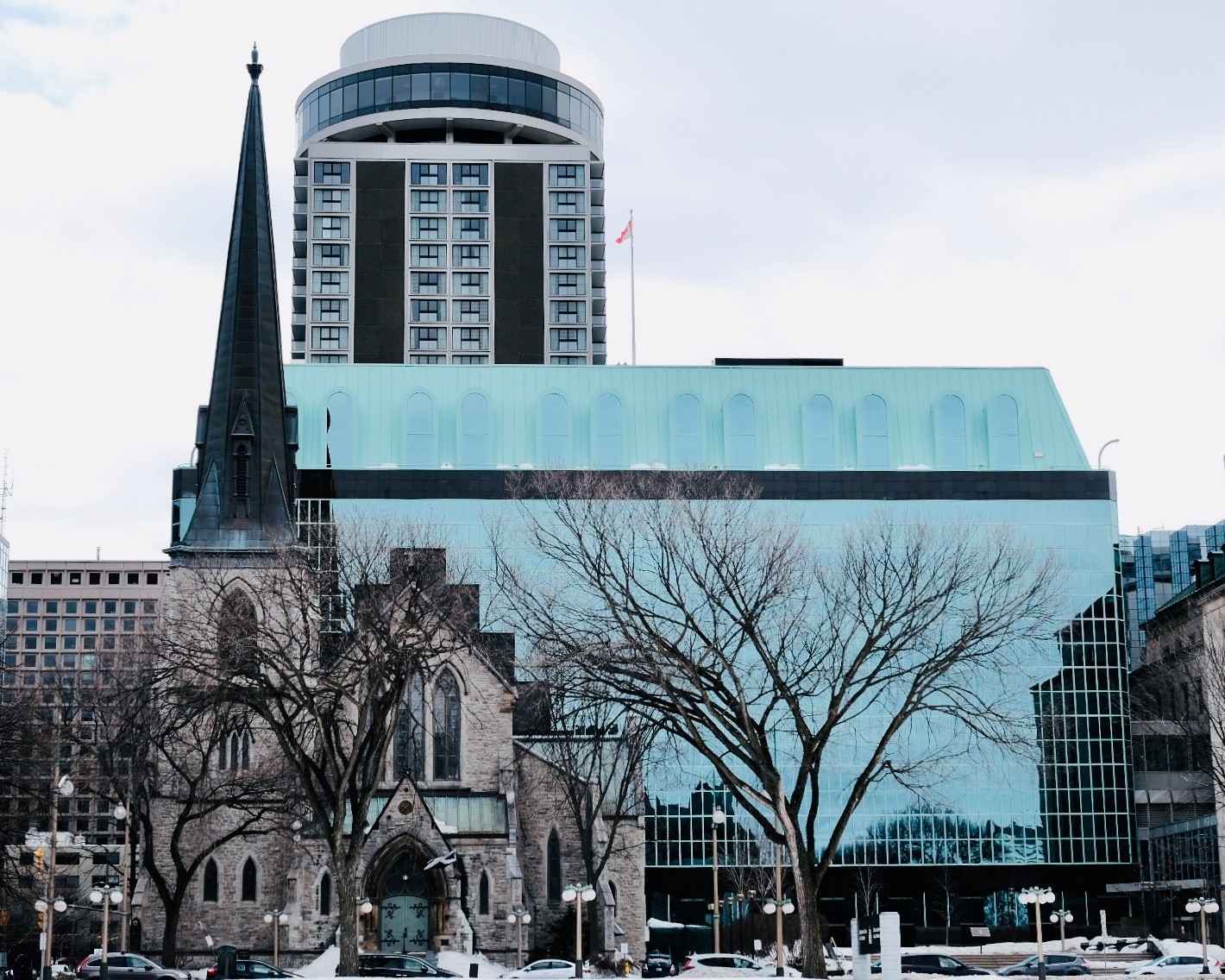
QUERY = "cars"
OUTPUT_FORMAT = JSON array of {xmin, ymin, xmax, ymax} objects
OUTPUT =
[
  {"xmin": 996, "ymin": 951, "xmax": 1093, "ymax": 977},
  {"xmin": 1123, "ymin": 952, "xmax": 1222, "ymax": 978},
  {"xmin": 638, "ymin": 953, "xmax": 680, "ymax": 978},
  {"xmin": 678, "ymin": 951, "xmax": 794, "ymax": 978},
  {"xmin": 496, "ymin": 958, "xmax": 593, "ymax": 980},
  {"xmin": 206, "ymin": 959, "xmax": 306, "ymax": 980},
  {"xmin": 822, "ymin": 945, "xmax": 999, "ymax": 976}
]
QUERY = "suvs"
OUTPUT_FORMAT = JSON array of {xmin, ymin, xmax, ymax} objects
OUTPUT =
[
  {"xmin": 75, "ymin": 951, "xmax": 192, "ymax": 980},
  {"xmin": 335, "ymin": 951, "xmax": 464, "ymax": 979}
]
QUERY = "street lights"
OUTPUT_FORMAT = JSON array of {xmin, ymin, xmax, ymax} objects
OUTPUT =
[
  {"xmin": 43, "ymin": 758, "xmax": 75, "ymax": 980},
  {"xmin": 34, "ymin": 895, "xmax": 68, "ymax": 980},
  {"xmin": 355, "ymin": 896, "xmax": 373, "ymax": 948},
  {"xmin": 711, "ymin": 804, "xmax": 726, "ymax": 953},
  {"xmin": 113, "ymin": 794, "xmax": 131, "ymax": 954},
  {"xmin": 1018, "ymin": 885, "xmax": 1056, "ymax": 980},
  {"xmin": 505, "ymin": 909, "xmax": 532, "ymax": 970},
  {"xmin": 262, "ymin": 909, "xmax": 290, "ymax": 968},
  {"xmin": 763, "ymin": 893, "xmax": 795, "ymax": 977},
  {"xmin": 1185, "ymin": 897, "xmax": 1220, "ymax": 974},
  {"xmin": 561, "ymin": 883, "xmax": 597, "ymax": 980},
  {"xmin": 1050, "ymin": 909, "xmax": 1074, "ymax": 952},
  {"xmin": 89, "ymin": 885, "xmax": 124, "ymax": 980}
]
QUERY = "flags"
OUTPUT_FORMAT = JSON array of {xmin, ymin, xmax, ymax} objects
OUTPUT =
[{"xmin": 615, "ymin": 219, "xmax": 633, "ymax": 244}]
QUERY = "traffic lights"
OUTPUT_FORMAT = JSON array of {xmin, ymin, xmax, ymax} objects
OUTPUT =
[
  {"xmin": 37, "ymin": 912, "xmax": 43, "ymax": 930},
  {"xmin": 33, "ymin": 846, "xmax": 43, "ymax": 872}
]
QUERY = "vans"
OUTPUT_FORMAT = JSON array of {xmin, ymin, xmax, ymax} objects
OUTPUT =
[{"xmin": 51, "ymin": 964, "xmax": 77, "ymax": 978}]
